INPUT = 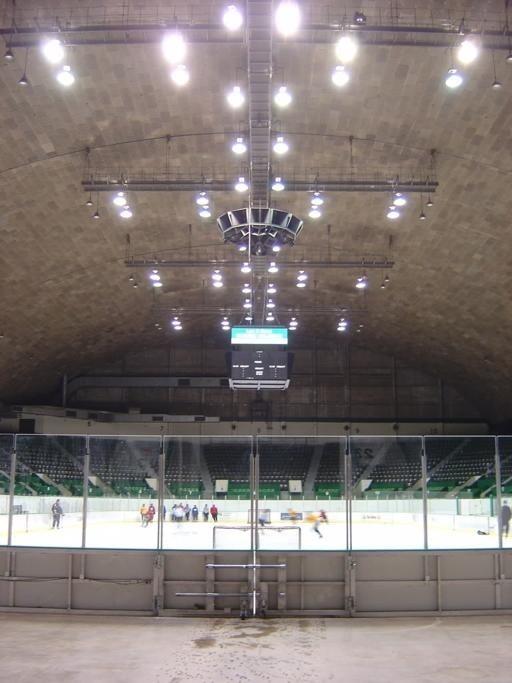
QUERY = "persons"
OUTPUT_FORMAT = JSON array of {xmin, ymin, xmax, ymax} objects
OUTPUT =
[
  {"xmin": 259, "ymin": 508, "xmax": 328, "ymax": 537},
  {"xmin": 52, "ymin": 499, "xmax": 63, "ymax": 528},
  {"xmin": 142, "ymin": 502, "xmax": 217, "ymax": 526},
  {"xmin": 500, "ymin": 500, "xmax": 512, "ymax": 536}
]
